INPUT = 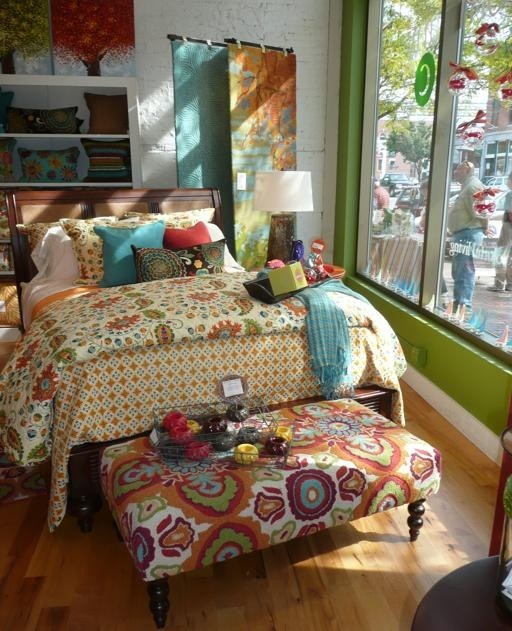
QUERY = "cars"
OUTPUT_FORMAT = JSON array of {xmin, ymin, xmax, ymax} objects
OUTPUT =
[
  {"xmin": 394, "ymin": 187, "xmax": 423, "ymax": 217},
  {"xmin": 480, "ymin": 175, "xmax": 508, "ymax": 222},
  {"xmin": 448, "ymin": 182, "xmax": 463, "ymax": 197}
]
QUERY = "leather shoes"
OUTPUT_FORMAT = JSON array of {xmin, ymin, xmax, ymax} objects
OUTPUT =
[{"xmin": 488, "ymin": 284, "xmax": 505, "ymax": 293}]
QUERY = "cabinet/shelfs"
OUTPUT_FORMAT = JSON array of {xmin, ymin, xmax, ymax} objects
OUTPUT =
[{"xmin": 0, "ymin": 72, "xmax": 142, "ymax": 187}]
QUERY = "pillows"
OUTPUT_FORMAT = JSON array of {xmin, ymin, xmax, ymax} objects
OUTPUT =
[
  {"xmin": 91, "ymin": 220, "xmax": 165, "ymax": 288},
  {"xmin": 15, "ymin": 214, "xmax": 119, "ymax": 286},
  {"xmin": 126, "ymin": 239, "xmax": 227, "ymax": 283},
  {"xmin": 163, "ymin": 222, "xmax": 212, "ymax": 249},
  {"xmin": 59, "ymin": 216, "xmax": 148, "ymax": 286},
  {"xmin": 125, "ymin": 208, "xmax": 218, "ymax": 282}
]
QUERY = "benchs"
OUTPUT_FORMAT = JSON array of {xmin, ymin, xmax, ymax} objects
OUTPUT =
[{"xmin": 97, "ymin": 397, "xmax": 442, "ymax": 627}]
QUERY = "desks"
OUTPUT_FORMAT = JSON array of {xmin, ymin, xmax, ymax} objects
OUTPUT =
[{"xmin": 413, "ymin": 553, "xmax": 512, "ymax": 630}]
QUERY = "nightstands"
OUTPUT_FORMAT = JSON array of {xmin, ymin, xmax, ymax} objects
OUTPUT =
[{"xmin": 249, "ymin": 260, "xmax": 345, "ymax": 302}]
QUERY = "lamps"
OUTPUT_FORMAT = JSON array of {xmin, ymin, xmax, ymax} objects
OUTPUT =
[{"xmin": 254, "ymin": 172, "xmax": 313, "ymax": 271}]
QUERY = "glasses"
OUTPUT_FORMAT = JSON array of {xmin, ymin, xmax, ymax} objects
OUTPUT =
[{"xmin": 463, "ymin": 160, "xmax": 470, "ymax": 169}]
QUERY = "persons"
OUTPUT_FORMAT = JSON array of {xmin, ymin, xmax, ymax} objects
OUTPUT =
[
  {"xmin": 415, "ymin": 181, "xmax": 430, "ymax": 234},
  {"xmin": 446, "ymin": 160, "xmax": 486, "ymax": 322},
  {"xmin": 372, "ymin": 181, "xmax": 390, "ymax": 211},
  {"xmin": 484, "ymin": 170, "xmax": 512, "ymax": 293}
]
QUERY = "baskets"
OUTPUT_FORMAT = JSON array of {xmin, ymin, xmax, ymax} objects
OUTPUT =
[{"xmin": 152, "ymin": 397, "xmax": 291, "ymax": 470}]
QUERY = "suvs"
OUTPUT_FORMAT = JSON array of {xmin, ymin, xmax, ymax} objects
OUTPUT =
[{"xmin": 380, "ymin": 173, "xmax": 413, "ymax": 197}]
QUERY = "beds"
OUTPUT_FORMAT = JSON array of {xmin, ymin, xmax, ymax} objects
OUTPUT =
[{"xmin": 0, "ymin": 187, "xmax": 397, "ymax": 534}]
